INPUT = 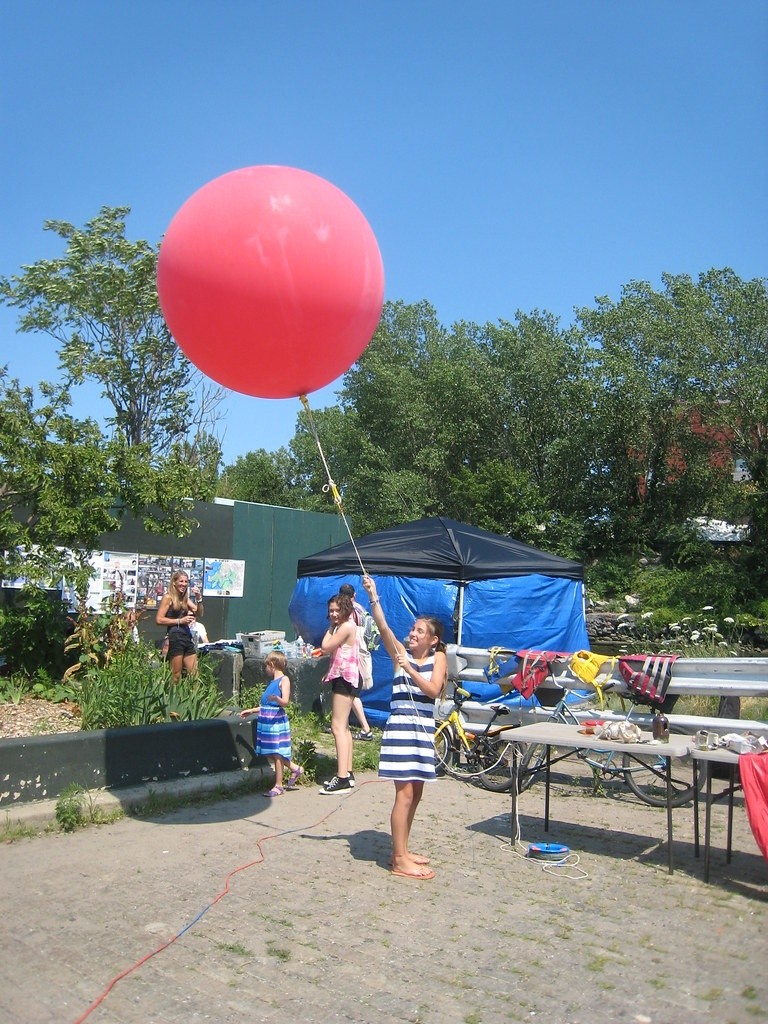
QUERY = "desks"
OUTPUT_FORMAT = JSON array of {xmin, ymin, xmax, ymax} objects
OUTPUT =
[
  {"xmin": 501, "ymin": 721, "xmax": 701, "ymax": 875},
  {"xmin": 690, "ymin": 743, "xmax": 768, "ymax": 884}
]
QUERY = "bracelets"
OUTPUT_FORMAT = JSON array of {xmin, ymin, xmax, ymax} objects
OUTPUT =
[
  {"xmin": 177, "ymin": 618, "xmax": 180, "ymax": 631},
  {"xmin": 195, "ymin": 597, "xmax": 202, "ymax": 604},
  {"xmin": 369, "ymin": 597, "xmax": 380, "ymax": 605}
]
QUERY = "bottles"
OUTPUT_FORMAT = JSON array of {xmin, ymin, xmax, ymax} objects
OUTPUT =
[
  {"xmin": 652, "ymin": 713, "xmax": 669, "ymax": 744},
  {"xmin": 306, "ymin": 643, "xmax": 311, "ymax": 660},
  {"xmin": 692, "ymin": 730, "xmax": 718, "ymax": 751},
  {"xmin": 296, "ymin": 636, "xmax": 303, "ymax": 659}
]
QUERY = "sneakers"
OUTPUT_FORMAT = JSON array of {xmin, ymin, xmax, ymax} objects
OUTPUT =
[{"xmin": 319, "ymin": 771, "xmax": 357, "ymax": 795}]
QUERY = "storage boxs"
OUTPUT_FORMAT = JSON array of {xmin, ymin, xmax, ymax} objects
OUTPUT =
[{"xmin": 240, "ymin": 631, "xmax": 286, "ymax": 658}]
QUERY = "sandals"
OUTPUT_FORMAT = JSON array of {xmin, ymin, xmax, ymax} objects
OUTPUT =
[
  {"xmin": 351, "ymin": 728, "xmax": 375, "ymax": 742},
  {"xmin": 287, "ymin": 765, "xmax": 305, "ymax": 787},
  {"xmin": 262, "ymin": 786, "xmax": 286, "ymax": 798}
]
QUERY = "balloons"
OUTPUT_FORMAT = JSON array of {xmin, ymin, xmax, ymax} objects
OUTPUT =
[{"xmin": 156, "ymin": 162, "xmax": 386, "ymax": 399}]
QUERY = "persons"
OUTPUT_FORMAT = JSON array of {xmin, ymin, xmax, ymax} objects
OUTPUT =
[
  {"xmin": 153, "ymin": 570, "xmax": 204, "ymax": 683},
  {"xmin": 318, "ymin": 593, "xmax": 363, "ymax": 796},
  {"xmin": 362, "ymin": 577, "xmax": 447, "ymax": 881},
  {"xmin": 319, "ymin": 582, "xmax": 374, "ymax": 742},
  {"xmin": 185, "ymin": 608, "xmax": 209, "ymax": 646},
  {"xmin": 239, "ymin": 650, "xmax": 304, "ymax": 797}
]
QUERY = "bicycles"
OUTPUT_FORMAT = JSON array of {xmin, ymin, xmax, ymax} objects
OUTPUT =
[
  {"xmin": 434, "ymin": 680, "xmax": 527, "ymax": 792},
  {"xmin": 518, "ymin": 661, "xmax": 708, "ymax": 808}
]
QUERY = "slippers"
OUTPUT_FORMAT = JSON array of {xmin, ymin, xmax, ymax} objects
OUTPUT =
[{"xmin": 388, "ymin": 853, "xmax": 438, "ymax": 881}]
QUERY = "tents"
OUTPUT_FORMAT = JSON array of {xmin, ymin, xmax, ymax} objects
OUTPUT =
[{"xmin": 287, "ymin": 516, "xmax": 595, "ymax": 731}]
